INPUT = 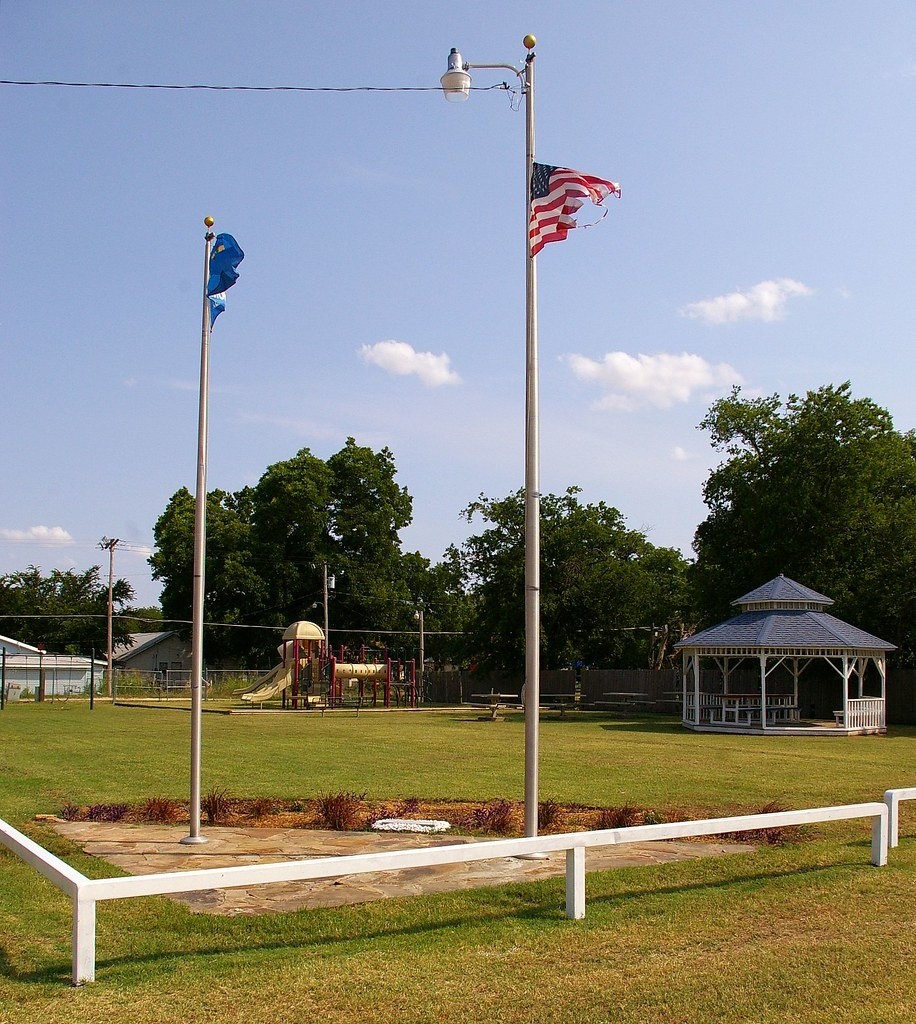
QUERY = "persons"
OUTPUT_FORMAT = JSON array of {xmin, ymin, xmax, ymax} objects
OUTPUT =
[{"xmin": 576, "ymin": 659, "xmax": 583, "ymax": 681}]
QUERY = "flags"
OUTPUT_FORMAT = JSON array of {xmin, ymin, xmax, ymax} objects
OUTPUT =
[
  {"xmin": 206, "ymin": 233, "xmax": 245, "ymax": 328},
  {"xmin": 528, "ymin": 162, "xmax": 622, "ymax": 258}
]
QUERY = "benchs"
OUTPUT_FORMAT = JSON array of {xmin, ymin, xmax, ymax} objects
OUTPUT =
[
  {"xmin": 656, "ymin": 699, "xmax": 692, "ymax": 704},
  {"xmin": 687, "ymin": 704, "xmax": 796, "ymax": 725},
  {"xmin": 594, "ymin": 700, "xmax": 656, "ymax": 706},
  {"xmin": 464, "ymin": 702, "xmax": 568, "ymax": 709},
  {"xmin": 834, "ymin": 706, "xmax": 881, "ymax": 727}
]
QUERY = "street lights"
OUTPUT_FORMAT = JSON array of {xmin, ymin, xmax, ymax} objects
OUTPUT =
[
  {"xmin": 414, "ymin": 597, "xmax": 426, "ymax": 702},
  {"xmin": 439, "ymin": 34, "xmax": 551, "ymax": 859}
]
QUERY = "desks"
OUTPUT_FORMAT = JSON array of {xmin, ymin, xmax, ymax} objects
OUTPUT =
[
  {"xmin": 656, "ymin": 691, "xmax": 703, "ymax": 715},
  {"xmin": 594, "ymin": 693, "xmax": 655, "ymax": 715},
  {"xmin": 464, "ymin": 693, "xmax": 586, "ymax": 722},
  {"xmin": 715, "ymin": 694, "xmax": 794, "ymax": 726}
]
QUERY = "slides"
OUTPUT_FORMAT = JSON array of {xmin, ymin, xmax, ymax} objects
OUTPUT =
[
  {"xmin": 232, "ymin": 660, "xmax": 284, "ymax": 695},
  {"xmin": 240, "ymin": 667, "xmax": 293, "ymax": 701}
]
